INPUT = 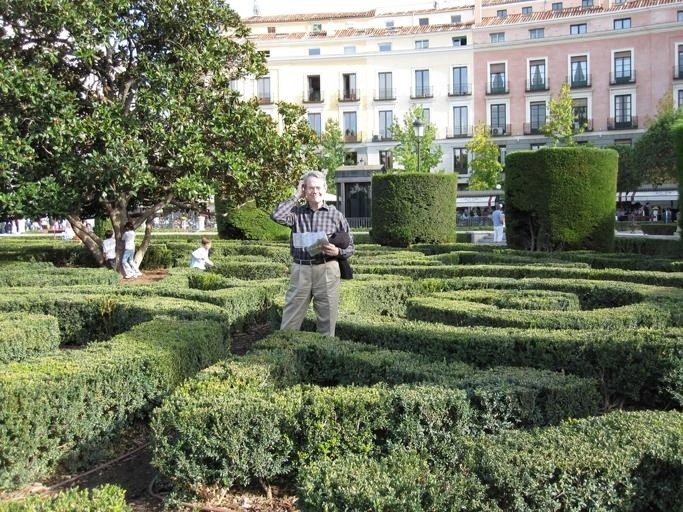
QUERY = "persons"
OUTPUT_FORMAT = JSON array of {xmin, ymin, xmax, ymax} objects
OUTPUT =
[
  {"xmin": 615, "ymin": 205, "xmax": 677, "ymax": 225},
  {"xmin": 492, "ymin": 203, "xmax": 504, "ymax": 243},
  {"xmin": 269, "ymin": 170, "xmax": 355, "ymax": 339},
  {"xmin": 23, "ymin": 217, "xmax": 92, "ymax": 240},
  {"xmin": 455, "ymin": 206, "xmax": 495, "ymax": 227},
  {"xmin": 154, "ymin": 213, "xmax": 217, "ymax": 230},
  {"xmin": 102, "ymin": 229, "xmax": 117, "ymax": 271},
  {"xmin": 190, "ymin": 238, "xmax": 214, "ymax": 272},
  {"xmin": 120, "ymin": 221, "xmax": 144, "ymax": 279}
]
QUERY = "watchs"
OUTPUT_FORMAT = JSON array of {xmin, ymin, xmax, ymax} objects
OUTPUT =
[{"xmin": 336, "ymin": 246, "xmax": 342, "ymax": 257}]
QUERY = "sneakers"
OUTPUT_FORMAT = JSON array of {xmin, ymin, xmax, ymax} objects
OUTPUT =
[{"xmin": 125, "ymin": 271, "xmax": 142, "ymax": 279}]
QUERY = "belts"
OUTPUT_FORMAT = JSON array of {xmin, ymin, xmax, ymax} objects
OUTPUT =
[{"xmin": 293, "ymin": 257, "xmax": 331, "ymax": 265}]
